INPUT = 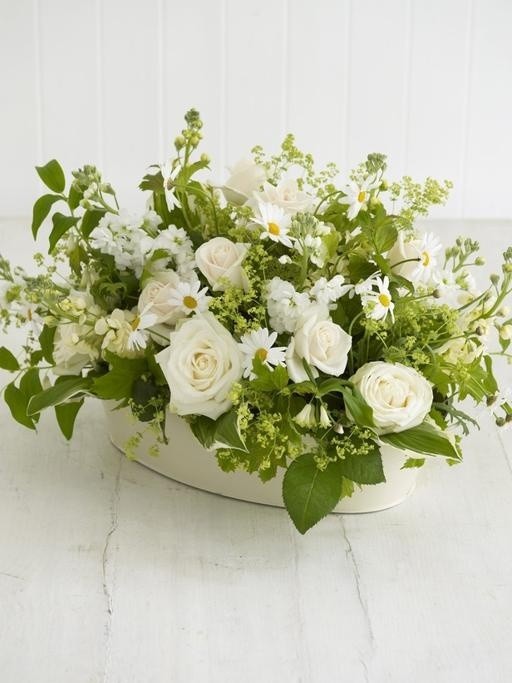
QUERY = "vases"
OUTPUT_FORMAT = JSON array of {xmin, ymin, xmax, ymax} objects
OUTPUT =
[{"xmin": 104, "ymin": 383, "xmax": 416, "ymax": 512}]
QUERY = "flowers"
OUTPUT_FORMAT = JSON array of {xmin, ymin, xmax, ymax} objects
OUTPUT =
[{"xmin": 0, "ymin": 107, "xmax": 512, "ymax": 535}]
